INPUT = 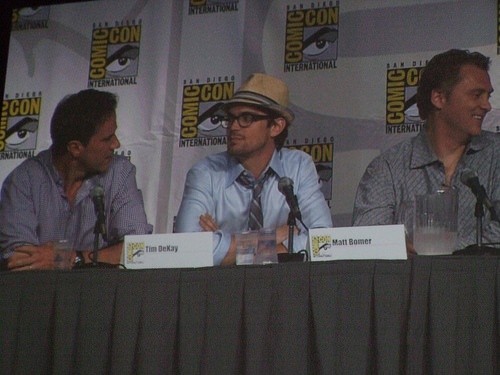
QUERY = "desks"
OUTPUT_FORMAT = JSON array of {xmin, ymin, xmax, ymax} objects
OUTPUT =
[{"xmin": 0, "ymin": 256, "xmax": 500, "ymax": 375}]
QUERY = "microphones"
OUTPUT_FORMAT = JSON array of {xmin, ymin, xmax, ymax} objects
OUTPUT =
[
  {"xmin": 461, "ymin": 168, "xmax": 496, "ymax": 216},
  {"xmin": 278, "ymin": 176, "xmax": 301, "ymax": 220},
  {"xmin": 89, "ymin": 186, "xmax": 108, "ymax": 240}
]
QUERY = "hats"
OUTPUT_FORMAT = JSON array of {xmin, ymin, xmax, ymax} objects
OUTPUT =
[{"xmin": 220, "ymin": 72, "xmax": 296, "ymax": 122}]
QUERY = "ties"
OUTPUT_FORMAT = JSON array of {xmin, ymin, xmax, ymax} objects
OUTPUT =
[{"xmin": 237, "ymin": 171, "xmax": 270, "ymax": 252}]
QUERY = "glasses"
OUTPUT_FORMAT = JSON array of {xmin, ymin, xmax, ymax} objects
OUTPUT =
[{"xmin": 221, "ymin": 111, "xmax": 279, "ymax": 129}]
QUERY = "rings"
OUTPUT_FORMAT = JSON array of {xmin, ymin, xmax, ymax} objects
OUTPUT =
[{"xmin": 30, "ymin": 265, "xmax": 31, "ymax": 270}]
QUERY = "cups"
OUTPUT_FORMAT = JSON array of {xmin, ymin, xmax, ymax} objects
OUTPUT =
[
  {"xmin": 398, "ymin": 188, "xmax": 460, "ymax": 256},
  {"xmin": 234, "ymin": 225, "xmax": 279, "ymax": 265},
  {"xmin": 54, "ymin": 239, "xmax": 71, "ymax": 270}
]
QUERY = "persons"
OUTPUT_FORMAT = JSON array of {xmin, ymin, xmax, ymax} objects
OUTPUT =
[
  {"xmin": 352, "ymin": 49, "xmax": 499, "ymax": 256},
  {"xmin": 0, "ymin": 90, "xmax": 153, "ymax": 272},
  {"xmin": 173, "ymin": 73, "xmax": 333, "ymax": 266}
]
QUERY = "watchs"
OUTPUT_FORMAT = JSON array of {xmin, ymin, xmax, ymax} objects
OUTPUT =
[{"xmin": 74, "ymin": 251, "xmax": 84, "ymax": 267}]
